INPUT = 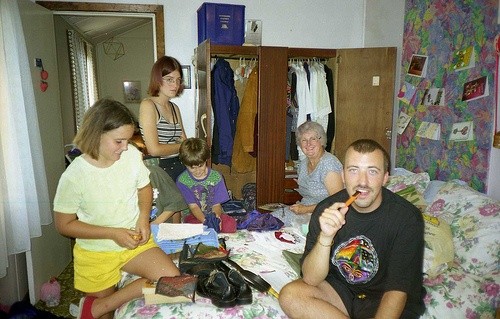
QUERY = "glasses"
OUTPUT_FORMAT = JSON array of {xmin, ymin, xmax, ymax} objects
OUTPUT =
[
  {"xmin": 299, "ymin": 137, "xmax": 321, "ymax": 146},
  {"xmin": 162, "ymin": 77, "xmax": 185, "ymax": 86}
]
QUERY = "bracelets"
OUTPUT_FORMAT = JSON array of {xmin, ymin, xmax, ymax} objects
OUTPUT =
[{"xmin": 318, "ymin": 235, "xmax": 334, "ymax": 247}]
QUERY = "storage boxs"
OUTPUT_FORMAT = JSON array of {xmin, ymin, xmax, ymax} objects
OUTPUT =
[{"xmin": 197, "ymin": 2, "xmax": 246, "ymax": 45}]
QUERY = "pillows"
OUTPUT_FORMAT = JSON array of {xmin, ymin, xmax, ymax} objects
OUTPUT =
[
  {"xmin": 386, "ymin": 168, "xmax": 430, "ymax": 199},
  {"xmin": 425, "ymin": 179, "xmax": 500, "ymax": 274}
]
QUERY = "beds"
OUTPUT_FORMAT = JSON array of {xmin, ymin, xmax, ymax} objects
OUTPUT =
[{"xmin": 106, "ymin": 168, "xmax": 500, "ymax": 319}]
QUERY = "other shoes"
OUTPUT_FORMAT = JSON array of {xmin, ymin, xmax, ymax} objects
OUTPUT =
[{"xmin": 77, "ymin": 296, "xmax": 97, "ymax": 319}]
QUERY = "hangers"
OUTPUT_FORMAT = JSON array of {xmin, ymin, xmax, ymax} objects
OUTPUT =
[
  {"xmin": 289, "ymin": 58, "xmax": 328, "ymax": 64},
  {"xmin": 240, "ymin": 57, "xmax": 257, "ymax": 68}
]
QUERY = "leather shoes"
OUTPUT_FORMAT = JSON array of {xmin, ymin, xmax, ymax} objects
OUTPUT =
[
  {"xmin": 216, "ymin": 264, "xmax": 252, "ymax": 305},
  {"xmin": 196, "ymin": 270, "xmax": 236, "ymax": 307}
]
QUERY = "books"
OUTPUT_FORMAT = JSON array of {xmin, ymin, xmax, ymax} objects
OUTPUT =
[{"xmin": 141, "ymin": 279, "xmax": 195, "ymax": 304}]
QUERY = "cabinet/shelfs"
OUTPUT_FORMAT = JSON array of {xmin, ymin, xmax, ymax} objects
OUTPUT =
[{"xmin": 193, "ymin": 38, "xmax": 397, "ymax": 209}]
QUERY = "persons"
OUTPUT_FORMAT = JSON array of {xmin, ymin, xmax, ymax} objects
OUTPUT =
[
  {"xmin": 271, "ymin": 122, "xmax": 344, "ymax": 226},
  {"xmin": 54, "ymin": 99, "xmax": 181, "ymax": 319},
  {"xmin": 279, "ymin": 140, "xmax": 426, "ymax": 319},
  {"xmin": 140, "ymin": 56, "xmax": 188, "ymax": 179},
  {"xmin": 174, "ymin": 138, "xmax": 230, "ymax": 230}
]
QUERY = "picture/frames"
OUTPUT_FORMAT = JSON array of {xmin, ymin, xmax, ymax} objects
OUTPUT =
[
  {"xmin": 122, "ymin": 80, "xmax": 141, "ymax": 103},
  {"xmin": 179, "ymin": 65, "xmax": 191, "ymax": 90}
]
